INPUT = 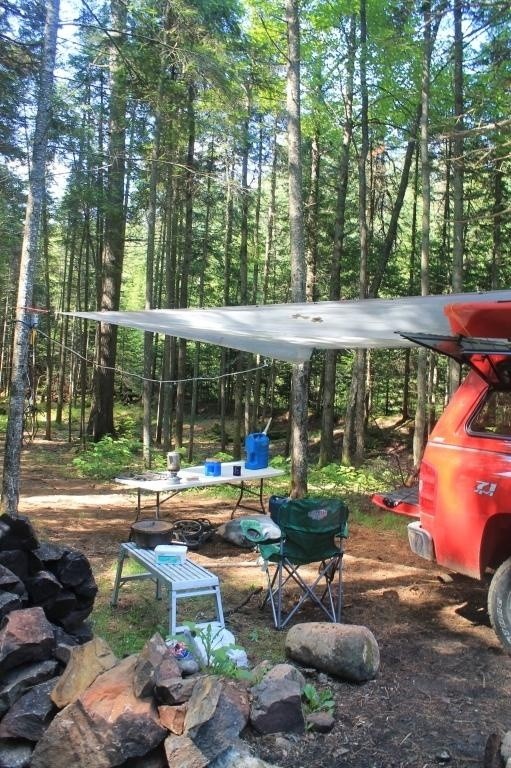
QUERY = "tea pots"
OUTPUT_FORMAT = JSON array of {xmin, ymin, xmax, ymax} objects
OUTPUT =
[{"xmin": 167, "ymin": 452, "xmax": 180, "ymax": 472}]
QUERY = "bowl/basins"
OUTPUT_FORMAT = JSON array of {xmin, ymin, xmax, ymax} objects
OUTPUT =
[{"xmin": 131, "ymin": 519, "xmax": 174, "ymax": 549}]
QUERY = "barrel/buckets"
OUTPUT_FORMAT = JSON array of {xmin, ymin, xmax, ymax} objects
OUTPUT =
[{"xmin": 245, "ymin": 432, "xmax": 270, "ymax": 470}]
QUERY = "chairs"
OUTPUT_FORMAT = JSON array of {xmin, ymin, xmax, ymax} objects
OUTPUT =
[{"xmin": 240, "ymin": 498, "xmax": 348, "ymax": 631}]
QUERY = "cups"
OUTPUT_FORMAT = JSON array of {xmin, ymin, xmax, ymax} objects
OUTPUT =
[{"xmin": 232, "ymin": 465, "xmax": 241, "ymax": 476}]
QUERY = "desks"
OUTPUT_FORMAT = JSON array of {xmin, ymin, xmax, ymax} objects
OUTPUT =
[
  {"xmin": 115, "ymin": 461, "xmax": 285, "ymax": 543},
  {"xmin": 111, "ymin": 541, "xmax": 226, "ymax": 636}
]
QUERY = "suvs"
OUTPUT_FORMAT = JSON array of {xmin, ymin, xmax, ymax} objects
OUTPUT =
[{"xmin": 371, "ymin": 299, "xmax": 511, "ymax": 661}]
